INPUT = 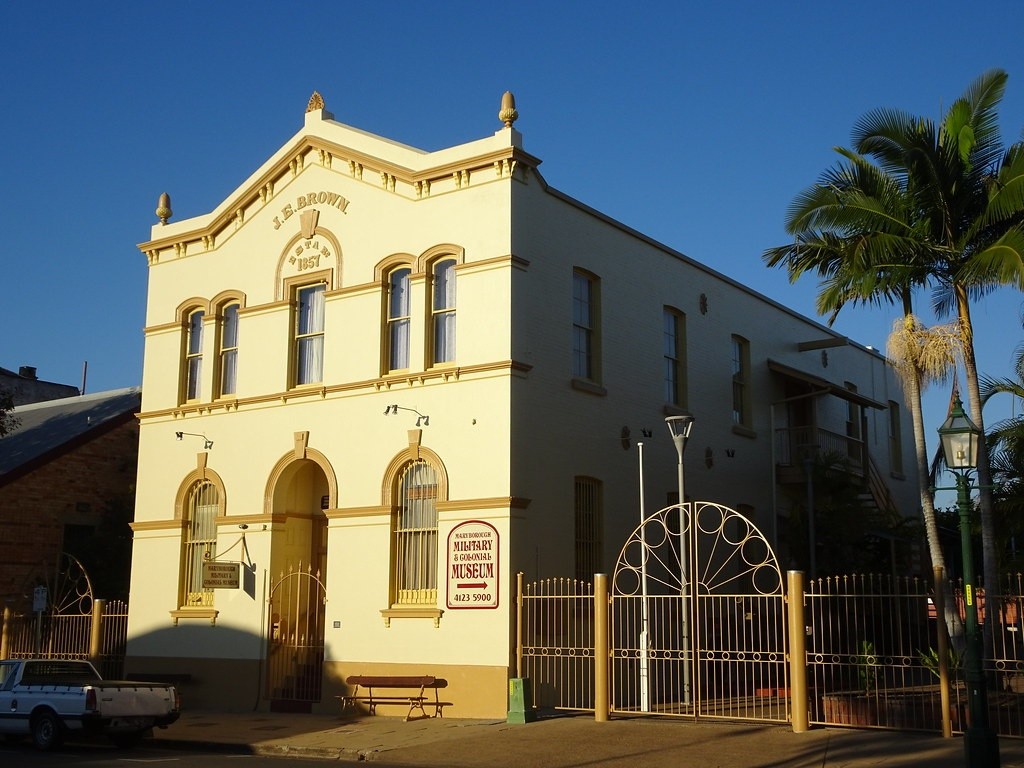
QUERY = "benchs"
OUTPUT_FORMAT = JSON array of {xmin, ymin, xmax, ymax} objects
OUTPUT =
[
  {"xmin": 126, "ymin": 673, "xmax": 193, "ymax": 688},
  {"xmin": 332, "ymin": 674, "xmax": 435, "ymax": 722}
]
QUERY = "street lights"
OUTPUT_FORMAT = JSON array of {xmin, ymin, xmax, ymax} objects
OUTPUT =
[
  {"xmin": 665, "ymin": 414, "xmax": 695, "ymax": 707},
  {"xmin": 937, "ymin": 389, "xmax": 1003, "ymax": 768}
]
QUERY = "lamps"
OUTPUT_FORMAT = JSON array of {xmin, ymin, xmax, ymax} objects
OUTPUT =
[
  {"xmin": 705, "ymin": 447, "xmax": 714, "ymax": 469},
  {"xmin": 622, "ymin": 426, "xmax": 631, "ymax": 450},
  {"xmin": 821, "ymin": 350, "xmax": 829, "ymax": 368},
  {"xmin": 175, "ymin": 432, "xmax": 208, "ymax": 441},
  {"xmin": 383, "ymin": 404, "xmax": 423, "ymax": 417},
  {"xmin": 700, "ymin": 294, "xmax": 708, "ymax": 315}
]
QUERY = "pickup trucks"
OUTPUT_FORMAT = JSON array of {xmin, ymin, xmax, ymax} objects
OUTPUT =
[{"xmin": 0, "ymin": 658, "xmax": 183, "ymax": 748}]
